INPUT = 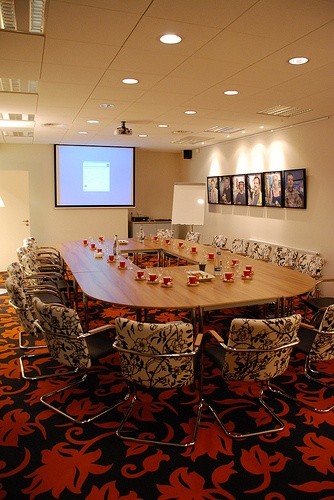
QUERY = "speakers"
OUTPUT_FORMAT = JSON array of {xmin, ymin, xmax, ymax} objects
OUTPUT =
[{"xmin": 184, "ymin": 149, "xmax": 192, "ymax": 159}]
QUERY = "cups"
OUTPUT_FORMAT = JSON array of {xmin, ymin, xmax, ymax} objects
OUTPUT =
[
  {"xmin": 209, "ymin": 253, "xmax": 214, "ymax": 259},
  {"xmin": 224, "ymin": 272, "xmax": 234, "ymax": 280},
  {"xmin": 154, "ymin": 236, "xmax": 157, "ymax": 240},
  {"xmin": 166, "ymin": 239, "xmax": 169, "ymax": 243},
  {"xmin": 91, "ymin": 244, "xmax": 95, "ymax": 249},
  {"xmin": 83, "ymin": 239, "xmax": 87, "ymax": 244},
  {"xmin": 198, "ymin": 261, "xmax": 206, "ymax": 272},
  {"xmin": 119, "ymin": 261, "xmax": 125, "ymax": 268},
  {"xmin": 98, "ymin": 249, "xmax": 102, "ymax": 253},
  {"xmin": 192, "ymin": 247, "xmax": 196, "ymax": 252},
  {"xmin": 242, "ymin": 265, "xmax": 252, "ymax": 278},
  {"xmin": 137, "ymin": 271, "xmax": 144, "ymax": 279},
  {"xmin": 163, "ymin": 276, "xmax": 174, "ymax": 286},
  {"xmin": 149, "ymin": 274, "xmax": 158, "ymax": 282},
  {"xmin": 232, "ymin": 259, "xmax": 239, "ymax": 266},
  {"xmin": 108, "ymin": 255, "xmax": 114, "ymax": 261},
  {"xmin": 179, "ymin": 242, "xmax": 183, "ymax": 247}
]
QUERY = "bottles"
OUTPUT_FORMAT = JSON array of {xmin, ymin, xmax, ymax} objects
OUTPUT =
[
  {"xmin": 113, "ymin": 235, "xmax": 118, "ymax": 256},
  {"xmin": 215, "ymin": 246, "xmax": 220, "ymax": 255},
  {"xmin": 214, "ymin": 252, "xmax": 223, "ymax": 276},
  {"xmin": 138, "ymin": 225, "xmax": 144, "ymax": 243}
]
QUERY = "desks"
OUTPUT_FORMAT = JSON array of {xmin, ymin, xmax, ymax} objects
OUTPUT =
[{"xmin": 58, "ymin": 230, "xmax": 317, "ymax": 339}]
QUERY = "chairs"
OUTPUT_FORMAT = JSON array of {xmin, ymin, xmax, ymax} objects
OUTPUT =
[{"xmin": 4, "ymin": 225, "xmax": 334, "ymax": 447}]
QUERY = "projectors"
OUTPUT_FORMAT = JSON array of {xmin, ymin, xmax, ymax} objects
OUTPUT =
[{"xmin": 114, "ymin": 127, "xmax": 133, "ymax": 135}]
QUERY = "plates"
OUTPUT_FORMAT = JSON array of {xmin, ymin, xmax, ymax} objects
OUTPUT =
[{"xmin": 186, "ymin": 270, "xmax": 216, "ymax": 282}]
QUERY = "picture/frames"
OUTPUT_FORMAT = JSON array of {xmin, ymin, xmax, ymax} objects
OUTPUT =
[{"xmin": 206, "ymin": 168, "xmax": 307, "ymax": 209}]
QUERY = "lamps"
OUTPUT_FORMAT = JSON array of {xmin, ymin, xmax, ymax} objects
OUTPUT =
[{"xmin": 115, "ymin": 121, "xmax": 132, "ymax": 136}]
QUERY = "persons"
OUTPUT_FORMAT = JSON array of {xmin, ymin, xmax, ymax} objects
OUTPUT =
[
  {"xmin": 249, "ymin": 177, "xmax": 262, "ymax": 206},
  {"xmin": 222, "ymin": 178, "xmax": 231, "ymax": 204},
  {"xmin": 235, "ymin": 181, "xmax": 246, "ymax": 204},
  {"xmin": 285, "ymin": 173, "xmax": 305, "ymax": 207},
  {"xmin": 266, "ymin": 176, "xmax": 281, "ymax": 206},
  {"xmin": 210, "ymin": 179, "xmax": 218, "ymax": 203}
]
